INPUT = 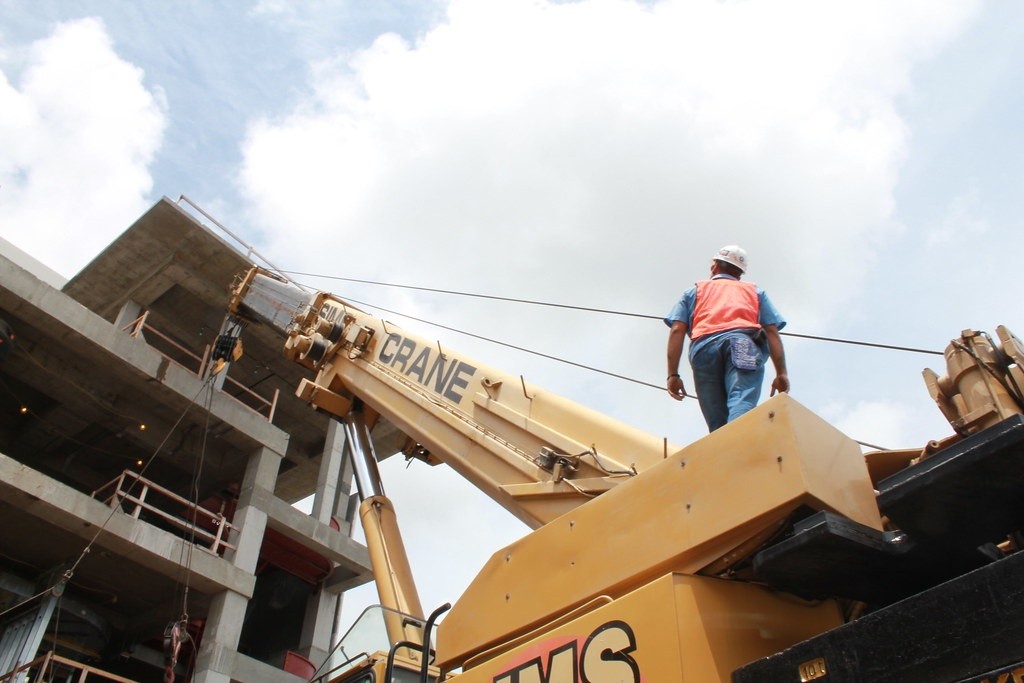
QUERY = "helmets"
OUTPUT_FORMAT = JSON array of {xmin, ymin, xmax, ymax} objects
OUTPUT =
[{"xmin": 712, "ymin": 244, "xmax": 750, "ymax": 274}]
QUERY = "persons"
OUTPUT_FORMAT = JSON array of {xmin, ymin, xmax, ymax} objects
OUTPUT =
[{"xmin": 662, "ymin": 243, "xmax": 791, "ymax": 435}]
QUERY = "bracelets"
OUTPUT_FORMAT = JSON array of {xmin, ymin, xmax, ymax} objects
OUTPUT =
[{"xmin": 667, "ymin": 374, "xmax": 680, "ymax": 380}]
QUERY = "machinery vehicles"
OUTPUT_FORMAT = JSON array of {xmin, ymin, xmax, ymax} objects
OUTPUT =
[{"xmin": 211, "ymin": 264, "xmax": 1024, "ymax": 683}]
